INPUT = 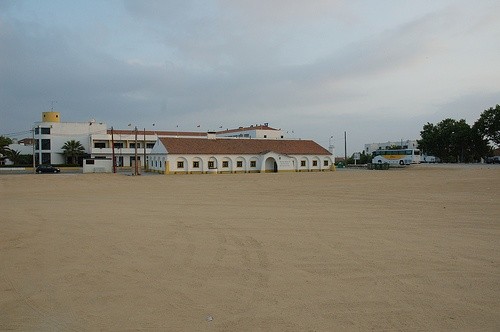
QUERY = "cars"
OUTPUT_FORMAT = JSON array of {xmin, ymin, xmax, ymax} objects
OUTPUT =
[{"xmin": 35, "ymin": 165, "xmax": 60, "ymax": 174}]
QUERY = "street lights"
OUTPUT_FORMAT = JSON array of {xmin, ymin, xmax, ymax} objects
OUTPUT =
[{"xmin": 329, "ymin": 136, "xmax": 333, "ymax": 145}]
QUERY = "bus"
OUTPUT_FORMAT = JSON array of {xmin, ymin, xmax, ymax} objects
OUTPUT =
[{"xmin": 371, "ymin": 148, "xmax": 421, "ymax": 165}]
